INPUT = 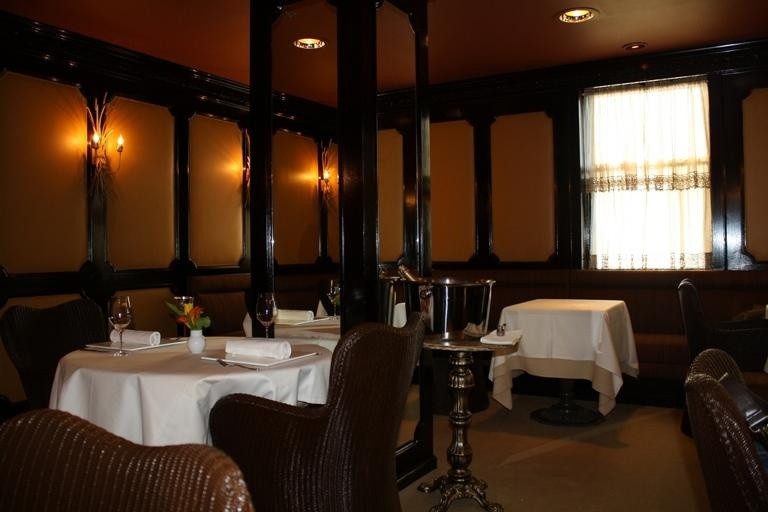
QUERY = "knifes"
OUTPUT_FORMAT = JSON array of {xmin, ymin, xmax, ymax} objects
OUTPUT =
[{"xmin": 80, "ymin": 348, "xmax": 110, "ymax": 353}]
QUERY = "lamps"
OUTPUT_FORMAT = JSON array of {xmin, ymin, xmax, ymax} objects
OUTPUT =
[
  {"xmin": 317, "ymin": 139, "xmax": 339, "ymax": 219},
  {"xmin": 553, "ymin": 7, "xmax": 599, "ymax": 25},
  {"xmin": 622, "ymin": 41, "xmax": 648, "ymax": 53},
  {"xmin": 293, "ymin": 38, "xmax": 325, "ymax": 50},
  {"xmin": 83, "ymin": 92, "xmax": 126, "ymax": 203}
]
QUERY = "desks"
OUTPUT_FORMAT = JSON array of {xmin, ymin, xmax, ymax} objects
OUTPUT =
[
  {"xmin": 272, "ymin": 303, "xmax": 341, "ymax": 355},
  {"xmin": 418, "ymin": 329, "xmax": 519, "ymax": 511},
  {"xmin": 488, "ymin": 299, "xmax": 640, "ymax": 428}
]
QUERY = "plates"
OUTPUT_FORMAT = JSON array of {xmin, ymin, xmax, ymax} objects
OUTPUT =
[
  {"xmin": 271, "ymin": 316, "xmax": 330, "ymax": 326},
  {"xmin": 86, "ymin": 337, "xmax": 187, "ymax": 352},
  {"xmin": 200, "ymin": 350, "xmax": 318, "ymax": 367}
]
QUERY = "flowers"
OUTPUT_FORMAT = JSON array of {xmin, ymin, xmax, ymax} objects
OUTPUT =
[{"xmin": 162, "ymin": 298, "xmax": 212, "ymax": 329}]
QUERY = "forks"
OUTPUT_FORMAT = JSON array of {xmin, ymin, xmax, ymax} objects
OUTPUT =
[{"xmin": 216, "ymin": 359, "xmax": 263, "ymax": 371}]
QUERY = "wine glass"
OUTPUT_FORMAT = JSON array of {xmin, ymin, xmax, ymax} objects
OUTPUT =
[
  {"xmin": 255, "ymin": 290, "xmax": 279, "ymax": 341},
  {"xmin": 107, "ymin": 295, "xmax": 133, "ymax": 357},
  {"xmin": 326, "ymin": 279, "xmax": 340, "ymax": 321}
]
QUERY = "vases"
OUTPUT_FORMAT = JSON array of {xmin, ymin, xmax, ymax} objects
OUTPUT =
[{"xmin": 188, "ymin": 329, "xmax": 208, "ymax": 355}]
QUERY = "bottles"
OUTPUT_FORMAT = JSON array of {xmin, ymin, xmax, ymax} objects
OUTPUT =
[{"xmin": 398, "ymin": 263, "xmax": 417, "ymax": 282}]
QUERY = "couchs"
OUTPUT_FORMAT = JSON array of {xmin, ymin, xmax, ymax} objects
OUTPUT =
[{"xmin": 2, "ymin": 274, "xmax": 768, "ymax": 405}]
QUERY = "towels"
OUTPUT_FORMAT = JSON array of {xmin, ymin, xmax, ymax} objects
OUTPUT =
[
  {"xmin": 225, "ymin": 340, "xmax": 292, "ymax": 359},
  {"xmin": 480, "ymin": 328, "xmax": 523, "ymax": 346},
  {"xmin": 110, "ymin": 328, "xmax": 161, "ymax": 346},
  {"xmin": 274, "ymin": 309, "xmax": 315, "ymax": 322}
]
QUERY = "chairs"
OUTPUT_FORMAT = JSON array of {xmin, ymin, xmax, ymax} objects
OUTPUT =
[
  {"xmin": 210, "ymin": 311, "xmax": 424, "ymax": 510},
  {"xmin": 678, "ymin": 278, "xmax": 765, "ymax": 434},
  {"xmin": 683, "ymin": 349, "xmax": 767, "ymax": 511},
  {"xmin": 2, "ymin": 407, "xmax": 254, "ymax": 511}
]
